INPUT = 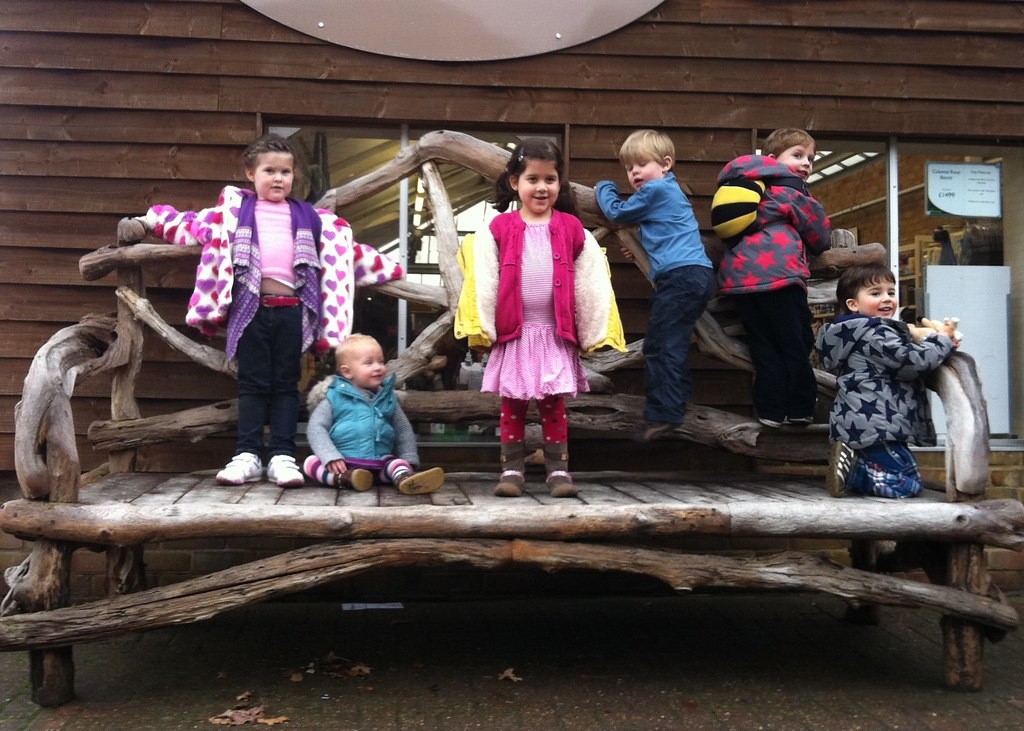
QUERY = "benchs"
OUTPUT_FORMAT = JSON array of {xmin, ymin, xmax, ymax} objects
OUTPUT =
[{"xmin": 0, "ymin": 130, "xmax": 1024, "ymax": 708}]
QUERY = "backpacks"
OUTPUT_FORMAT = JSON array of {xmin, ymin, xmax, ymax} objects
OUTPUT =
[{"xmin": 710, "ymin": 173, "xmax": 809, "ymax": 247}]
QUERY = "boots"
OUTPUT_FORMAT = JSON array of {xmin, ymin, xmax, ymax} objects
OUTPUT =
[
  {"xmin": 541, "ymin": 443, "xmax": 579, "ymax": 498},
  {"xmin": 493, "ymin": 439, "xmax": 527, "ymax": 497}
]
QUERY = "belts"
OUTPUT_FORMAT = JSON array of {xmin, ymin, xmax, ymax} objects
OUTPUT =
[{"xmin": 258, "ymin": 294, "xmax": 301, "ymax": 307}]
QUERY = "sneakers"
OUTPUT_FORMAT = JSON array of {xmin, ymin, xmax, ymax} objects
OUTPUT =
[
  {"xmin": 266, "ymin": 455, "xmax": 305, "ymax": 488},
  {"xmin": 826, "ymin": 441, "xmax": 855, "ymax": 497},
  {"xmin": 215, "ymin": 452, "xmax": 263, "ymax": 486}
]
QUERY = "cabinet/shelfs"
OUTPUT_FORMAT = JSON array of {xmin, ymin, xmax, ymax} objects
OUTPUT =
[{"xmin": 809, "ymin": 232, "xmax": 965, "ymax": 370}]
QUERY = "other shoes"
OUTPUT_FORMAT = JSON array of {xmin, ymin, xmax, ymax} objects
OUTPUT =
[
  {"xmin": 340, "ymin": 468, "xmax": 373, "ymax": 491},
  {"xmin": 757, "ymin": 416, "xmax": 783, "ymax": 429},
  {"xmin": 397, "ymin": 466, "xmax": 444, "ymax": 495},
  {"xmin": 641, "ymin": 419, "xmax": 680, "ymax": 442},
  {"xmin": 786, "ymin": 414, "xmax": 814, "ymax": 424}
]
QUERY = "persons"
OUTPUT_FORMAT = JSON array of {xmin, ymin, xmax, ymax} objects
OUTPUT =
[
  {"xmin": 711, "ymin": 127, "xmax": 831, "ymax": 427},
  {"xmin": 454, "ymin": 137, "xmax": 628, "ymax": 497},
  {"xmin": 815, "ymin": 263, "xmax": 962, "ymax": 499},
  {"xmin": 303, "ymin": 335, "xmax": 444, "ymax": 494},
  {"xmin": 594, "ymin": 129, "xmax": 717, "ymax": 442},
  {"xmin": 130, "ymin": 132, "xmax": 402, "ymax": 486}
]
